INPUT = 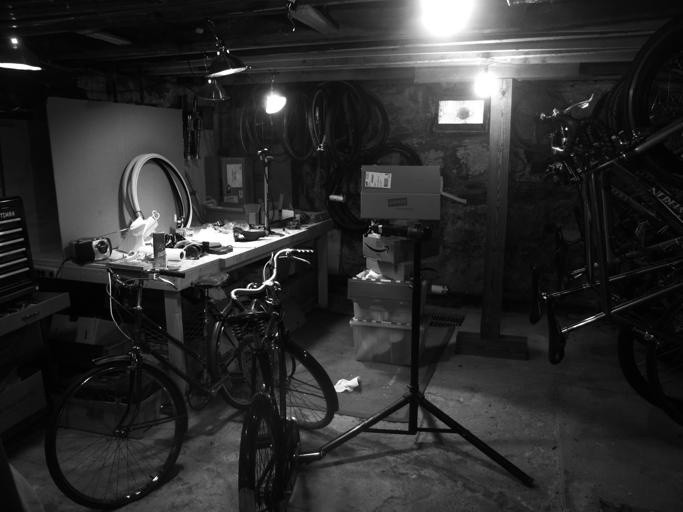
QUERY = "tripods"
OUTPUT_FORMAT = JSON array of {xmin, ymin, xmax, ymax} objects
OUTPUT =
[{"xmin": 306, "ymin": 227, "xmax": 533, "ymax": 491}]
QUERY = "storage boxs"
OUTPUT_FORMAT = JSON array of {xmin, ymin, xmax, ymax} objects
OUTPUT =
[
  {"xmin": 51, "ymin": 387, "xmax": 164, "ymax": 439},
  {"xmin": 348, "ymin": 163, "xmax": 468, "ymax": 366},
  {"xmin": 0, "ymin": 368, "xmax": 48, "ymax": 434}
]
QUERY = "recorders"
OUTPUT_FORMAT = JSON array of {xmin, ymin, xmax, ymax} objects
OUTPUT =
[{"xmin": 67, "ymin": 236, "xmax": 113, "ymax": 265}]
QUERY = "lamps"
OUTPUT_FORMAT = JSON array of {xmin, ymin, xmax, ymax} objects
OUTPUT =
[
  {"xmin": 265, "ymin": 79, "xmax": 287, "ymax": 114},
  {"xmin": 0, "ymin": 4, "xmax": 45, "ymax": 73},
  {"xmin": 194, "ymin": 26, "xmax": 249, "ymax": 79}
]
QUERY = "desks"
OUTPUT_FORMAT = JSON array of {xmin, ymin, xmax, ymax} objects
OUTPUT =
[{"xmin": 32, "ymin": 208, "xmax": 333, "ymax": 418}]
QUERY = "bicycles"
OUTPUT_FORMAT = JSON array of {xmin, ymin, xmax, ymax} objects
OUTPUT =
[
  {"xmin": 41, "ymin": 261, "xmax": 271, "ymax": 511},
  {"xmin": 221, "ymin": 245, "xmax": 340, "ymax": 512}
]
